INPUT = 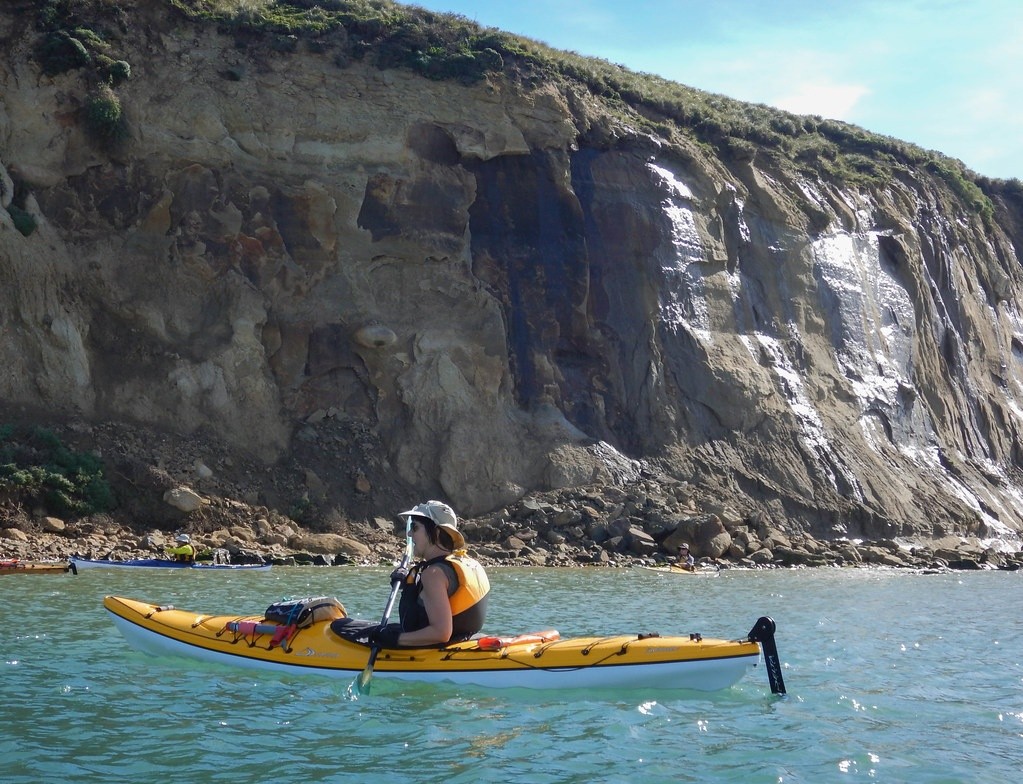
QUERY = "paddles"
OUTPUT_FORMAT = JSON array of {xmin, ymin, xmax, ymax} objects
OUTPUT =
[
  {"xmin": 349, "ymin": 505, "xmax": 418, "ymax": 699},
  {"xmin": 653, "ymin": 562, "xmax": 707, "ymax": 568},
  {"xmin": 145, "ymin": 535, "xmax": 169, "ymax": 560}
]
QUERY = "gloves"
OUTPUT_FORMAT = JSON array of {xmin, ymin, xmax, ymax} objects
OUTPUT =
[
  {"xmin": 389, "ymin": 567, "xmax": 410, "ymax": 590},
  {"xmin": 157, "ymin": 547, "xmax": 165, "ymax": 553},
  {"xmin": 373, "ymin": 624, "xmax": 402, "ymax": 648}
]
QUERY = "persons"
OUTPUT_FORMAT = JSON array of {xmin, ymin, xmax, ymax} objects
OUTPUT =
[
  {"xmin": 373, "ymin": 501, "xmax": 488, "ymax": 648},
  {"xmin": 156, "ymin": 533, "xmax": 196, "ymax": 562}
]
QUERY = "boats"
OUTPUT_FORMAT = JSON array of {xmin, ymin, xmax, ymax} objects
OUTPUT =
[
  {"xmin": 630, "ymin": 563, "xmax": 723, "ymax": 579},
  {"xmin": 0, "ymin": 562, "xmax": 77, "ymax": 576},
  {"xmin": 66, "ymin": 555, "xmax": 275, "ymax": 576},
  {"xmin": 103, "ymin": 593, "xmax": 787, "ymax": 698}
]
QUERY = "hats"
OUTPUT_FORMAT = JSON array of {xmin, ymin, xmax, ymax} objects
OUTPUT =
[
  {"xmin": 397, "ymin": 500, "xmax": 465, "ymax": 550},
  {"xmin": 176, "ymin": 534, "xmax": 191, "ymax": 543}
]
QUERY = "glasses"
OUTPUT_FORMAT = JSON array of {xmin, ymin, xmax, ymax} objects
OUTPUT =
[{"xmin": 410, "ymin": 522, "xmax": 428, "ymax": 532}]
